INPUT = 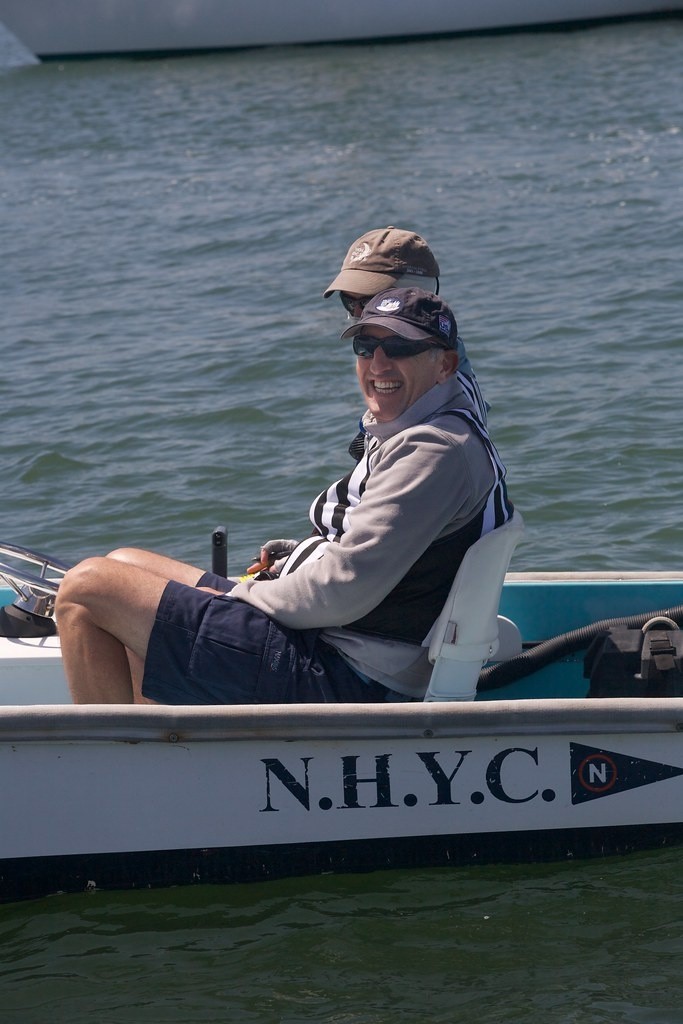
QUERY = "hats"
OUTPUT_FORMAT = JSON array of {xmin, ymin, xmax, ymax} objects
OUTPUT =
[
  {"xmin": 323, "ymin": 226, "xmax": 439, "ymax": 299},
  {"xmin": 340, "ymin": 286, "xmax": 458, "ymax": 351}
]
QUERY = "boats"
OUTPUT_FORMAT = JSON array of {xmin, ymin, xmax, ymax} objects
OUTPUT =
[
  {"xmin": 0, "ymin": 1, "xmax": 682, "ymax": 62},
  {"xmin": 0, "ymin": 528, "xmax": 683, "ymax": 898}
]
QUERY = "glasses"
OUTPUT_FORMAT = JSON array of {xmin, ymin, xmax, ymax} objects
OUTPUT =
[
  {"xmin": 339, "ymin": 291, "xmax": 375, "ymax": 318},
  {"xmin": 351, "ymin": 333, "xmax": 447, "ymax": 359}
]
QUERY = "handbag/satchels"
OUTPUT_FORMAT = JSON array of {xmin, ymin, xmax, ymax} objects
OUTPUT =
[{"xmin": 583, "ymin": 626, "xmax": 683, "ymax": 698}]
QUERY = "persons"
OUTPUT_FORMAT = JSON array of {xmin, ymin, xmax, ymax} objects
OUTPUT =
[
  {"xmin": 246, "ymin": 225, "xmax": 490, "ymax": 578},
  {"xmin": 53, "ymin": 286, "xmax": 514, "ymax": 704}
]
